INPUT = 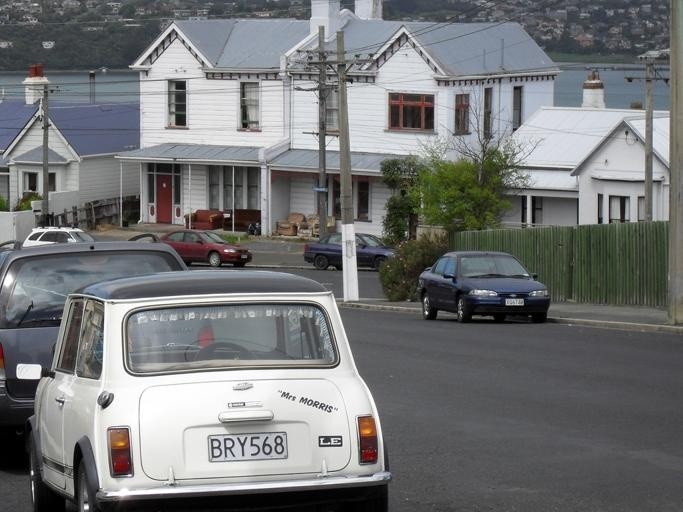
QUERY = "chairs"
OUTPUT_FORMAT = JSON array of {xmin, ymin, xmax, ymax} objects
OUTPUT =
[{"xmin": 275, "ymin": 213, "xmax": 336, "ymax": 238}]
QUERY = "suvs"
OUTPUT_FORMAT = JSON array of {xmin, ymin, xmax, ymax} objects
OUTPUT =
[{"xmin": 0, "ymin": 232, "xmax": 189, "ymax": 473}]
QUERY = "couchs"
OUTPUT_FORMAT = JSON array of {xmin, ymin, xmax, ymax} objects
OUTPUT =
[{"xmin": 183, "ymin": 208, "xmax": 260, "ymax": 232}]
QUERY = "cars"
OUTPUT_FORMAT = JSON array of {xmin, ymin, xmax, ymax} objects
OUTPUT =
[
  {"xmin": 417, "ymin": 250, "xmax": 550, "ymax": 323},
  {"xmin": 158, "ymin": 228, "xmax": 252, "ymax": 266},
  {"xmin": 303, "ymin": 234, "xmax": 403, "ymax": 273},
  {"xmin": 21, "ymin": 223, "xmax": 98, "ymax": 252},
  {"xmin": 16, "ymin": 270, "xmax": 389, "ymax": 512}
]
